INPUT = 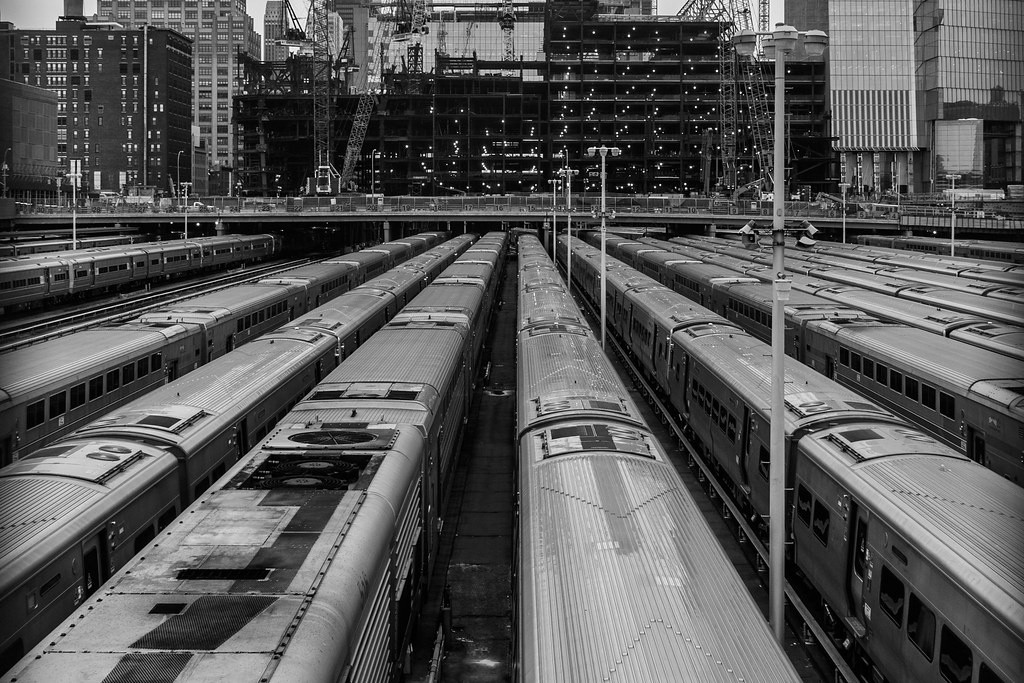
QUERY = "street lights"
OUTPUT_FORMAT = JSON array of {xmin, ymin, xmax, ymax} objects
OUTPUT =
[
  {"xmin": 371, "ymin": 149, "xmax": 378, "ymax": 207},
  {"xmin": 584, "ymin": 145, "xmax": 620, "ymax": 352},
  {"xmin": 179, "ymin": 181, "xmax": 193, "ymax": 239},
  {"xmin": 944, "ymin": 173, "xmax": 962, "ymax": 259},
  {"xmin": 65, "ymin": 174, "xmax": 82, "ymax": 249},
  {"xmin": 557, "ymin": 170, "xmax": 579, "ymax": 291},
  {"xmin": 837, "ymin": 183, "xmax": 852, "ymax": 242},
  {"xmin": 729, "ymin": 21, "xmax": 830, "ymax": 653},
  {"xmin": 176, "ymin": 151, "xmax": 185, "ymax": 210},
  {"xmin": 547, "ymin": 179, "xmax": 561, "ymax": 267}
]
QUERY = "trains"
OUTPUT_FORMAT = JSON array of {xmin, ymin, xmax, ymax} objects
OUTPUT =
[{"xmin": 0, "ymin": 218, "xmax": 1022, "ymax": 683}]
